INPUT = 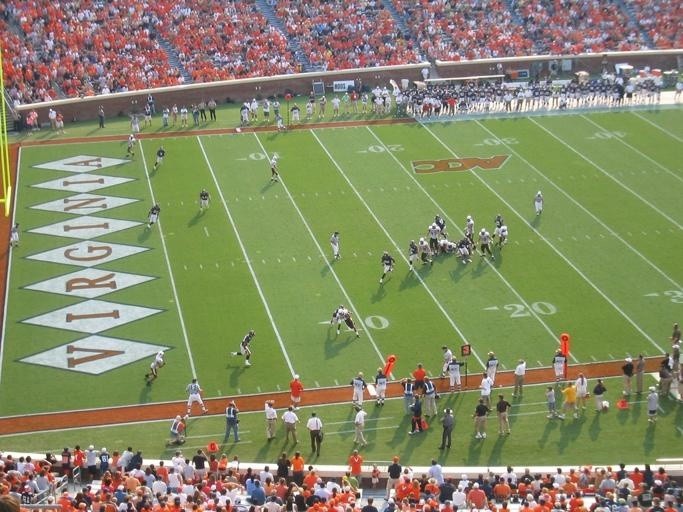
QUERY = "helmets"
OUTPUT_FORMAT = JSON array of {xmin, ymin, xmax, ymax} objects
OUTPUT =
[
  {"xmin": 410, "ymin": 237, "xmax": 425, "ymax": 243},
  {"xmin": 430, "ymin": 213, "xmax": 502, "ymax": 235},
  {"xmin": 384, "ymin": 250, "xmax": 389, "ymax": 256}
]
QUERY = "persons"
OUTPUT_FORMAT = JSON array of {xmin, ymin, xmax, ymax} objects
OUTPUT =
[
  {"xmin": 330, "ymin": 231, "xmax": 342, "ymax": 259},
  {"xmin": 621, "ymin": 323, "xmax": 682, "ymax": 423},
  {"xmin": 231, "ymin": 329, "xmax": 255, "ymax": 366},
  {"xmin": 399, "ymin": 346, "xmax": 462, "ymax": 449},
  {"xmin": 146, "ymin": 202, "xmax": 160, "ymax": 229},
  {"xmin": 380, "ymin": 252, "xmax": 396, "ymax": 284},
  {"xmin": 474, "ymin": 351, "xmax": 526, "ymax": 439},
  {"xmin": 384, "ymin": 457, "xmax": 682, "ymax": 511},
  {"xmin": 330, "ymin": 306, "xmax": 359, "ymax": 338},
  {"xmin": 153, "ymin": 146, "xmax": 165, "ymax": 170},
  {"xmin": 199, "ymin": 189, "xmax": 211, "ymax": 213},
  {"xmin": 0, "ymin": 0, "xmax": 303, "ymax": 132},
  {"xmin": 144, "ymin": 350, "xmax": 241, "ymax": 445},
  {"xmin": 265, "ymin": 375, "xmax": 323, "ymax": 457},
  {"xmin": 408, "ymin": 215, "xmax": 508, "ymax": 271},
  {"xmin": 9, "ymin": 222, "xmax": 22, "ymax": 248},
  {"xmin": 545, "ymin": 349, "xmax": 608, "ymax": 419},
  {"xmin": 0, "ymin": 442, "xmax": 382, "ymax": 512},
  {"xmin": 125, "ymin": 135, "xmax": 136, "ymax": 158},
  {"xmin": 510, "ymin": 1, "xmax": 682, "ymax": 110},
  {"xmin": 350, "ymin": 366, "xmax": 386, "ymax": 448},
  {"xmin": 533, "ymin": 191, "xmax": 545, "ymax": 217},
  {"xmin": 270, "ymin": 0, "xmax": 539, "ymax": 119},
  {"xmin": 269, "ymin": 156, "xmax": 278, "ymax": 183}
]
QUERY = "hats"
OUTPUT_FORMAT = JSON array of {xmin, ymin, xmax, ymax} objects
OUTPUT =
[
  {"xmin": 428, "ymin": 478, "xmax": 437, "ymax": 485},
  {"xmin": 625, "ymin": 358, "xmax": 632, "ymax": 363},
  {"xmin": 444, "ymin": 408, "xmax": 453, "ymax": 413},
  {"xmin": 654, "ymin": 479, "xmax": 662, "ymax": 486},
  {"xmin": 649, "ymin": 387, "xmax": 655, "ymax": 392}
]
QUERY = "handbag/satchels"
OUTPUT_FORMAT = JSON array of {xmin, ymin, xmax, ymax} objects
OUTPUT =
[{"xmin": 319, "ymin": 432, "xmax": 323, "ymax": 441}]
